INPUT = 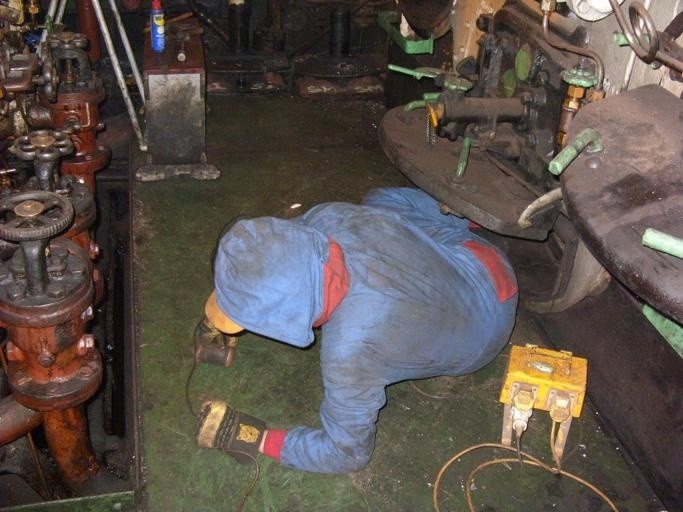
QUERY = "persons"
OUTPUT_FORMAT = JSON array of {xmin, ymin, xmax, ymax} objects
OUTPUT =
[{"xmin": 193, "ymin": 180, "xmax": 519, "ymax": 479}]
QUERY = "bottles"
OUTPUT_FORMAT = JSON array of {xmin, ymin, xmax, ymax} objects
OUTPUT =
[{"xmin": 149, "ymin": 1, "xmax": 165, "ymax": 54}]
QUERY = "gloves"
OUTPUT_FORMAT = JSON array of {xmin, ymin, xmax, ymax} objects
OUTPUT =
[
  {"xmin": 194, "ymin": 316, "xmax": 238, "ymax": 348},
  {"xmin": 193, "ymin": 400, "xmax": 266, "ymax": 465}
]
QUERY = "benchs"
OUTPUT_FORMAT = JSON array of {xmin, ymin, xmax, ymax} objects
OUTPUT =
[{"xmin": 141, "ymin": 13, "xmax": 210, "ymax": 165}]
